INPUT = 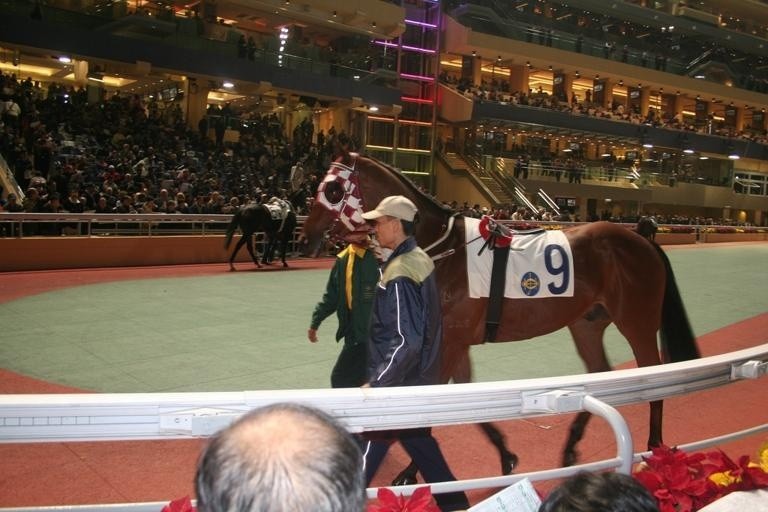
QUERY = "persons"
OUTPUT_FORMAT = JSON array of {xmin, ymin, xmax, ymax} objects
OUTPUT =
[
  {"xmin": 538, "ymin": 470, "xmax": 661, "ymax": 511},
  {"xmin": 439, "ymin": 1, "xmax": 768, "ymax": 232},
  {"xmin": 1, "ymin": 0, "xmax": 395, "ymax": 256},
  {"xmin": 309, "ymin": 233, "xmax": 393, "ymax": 388},
  {"xmin": 191, "ymin": 402, "xmax": 367, "ymax": 512},
  {"xmin": 358, "ymin": 194, "xmax": 472, "ymax": 512}
]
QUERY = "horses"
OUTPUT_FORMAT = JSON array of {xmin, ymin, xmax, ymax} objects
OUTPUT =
[
  {"xmin": 223, "ymin": 195, "xmax": 296, "ymax": 272},
  {"xmin": 636, "ymin": 216, "xmax": 658, "ymax": 240},
  {"xmin": 294, "ymin": 139, "xmax": 702, "ymax": 486}
]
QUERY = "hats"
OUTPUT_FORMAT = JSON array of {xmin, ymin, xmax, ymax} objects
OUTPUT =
[{"xmin": 361, "ymin": 195, "xmax": 420, "ymax": 223}]
